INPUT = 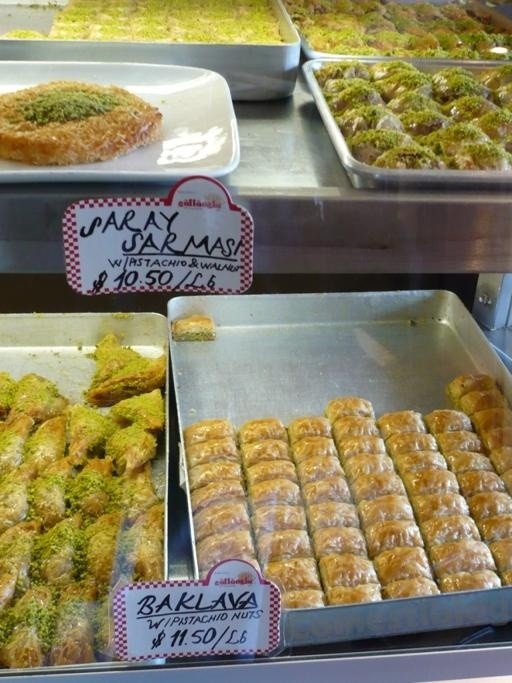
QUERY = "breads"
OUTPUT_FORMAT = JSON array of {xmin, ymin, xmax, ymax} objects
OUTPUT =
[
  {"xmin": 1, "ymin": 333, "xmax": 166, "ymax": 669},
  {"xmin": 171, "ymin": 314, "xmax": 215, "ymax": 343},
  {"xmin": 2, "ymin": 2, "xmax": 284, "ymax": 44},
  {"xmin": 0, "ymin": 78, "xmax": 161, "ymax": 167},
  {"xmin": 283, "ymin": 1, "xmax": 511, "ymax": 60},
  {"xmin": 182, "ymin": 373, "xmax": 512, "ymax": 669},
  {"xmin": 314, "ymin": 60, "xmax": 512, "ymax": 168}
]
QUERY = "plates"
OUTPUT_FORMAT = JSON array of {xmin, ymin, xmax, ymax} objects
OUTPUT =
[{"xmin": 1, "ymin": 59, "xmax": 241, "ymax": 182}]
